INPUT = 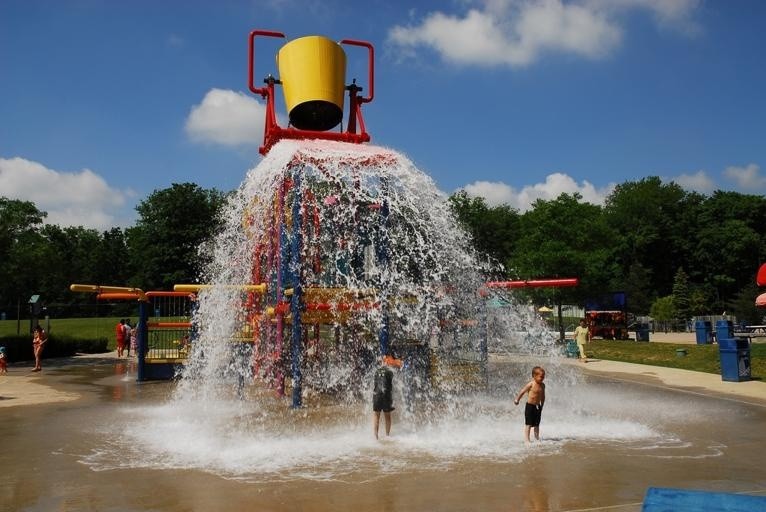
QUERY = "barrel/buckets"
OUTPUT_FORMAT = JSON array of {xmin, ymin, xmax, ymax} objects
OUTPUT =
[{"xmin": 274, "ymin": 34, "xmax": 348, "ymax": 132}]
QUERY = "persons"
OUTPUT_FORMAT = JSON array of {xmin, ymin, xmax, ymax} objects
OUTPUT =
[
  {"xmin": 573, "ymin": 318, "xmax": 591, "ymax": 359},
  {"xmin": 31, "ymin": 325, "xmax": 48, "ymax": 372},
  {"xmin": 0, "ymin": 346, "xmax": 8, "ymax": 375},
  {"xmin": 372, "ymin": 316, "xmax": 408, "ymax": 439},
  {"xmin": 688, "ymin": 318, "xmax": 693, "ymax": 332},
  {"xmin": 116, "ymin": 319, "xmax": 139, "ymax": 357},
  {"xmin": 514, "ymin": 366, "xmax": 545, "ymax": 446}
]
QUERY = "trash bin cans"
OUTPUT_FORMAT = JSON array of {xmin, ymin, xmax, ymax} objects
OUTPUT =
[
  {"xmin": 694, "ymin": 320, "xmax": 713, "ymax": 344},
  {"xmin": 718, "ymin": 339, "xmax": 751, "ymax": 382},
  {"xmin": 634, "ymin": 323, "xmax": 649, "ymax": 342},
  {"xmin": 716, "ymin": 320, "xmax": 734, "ymax": 344}
]
